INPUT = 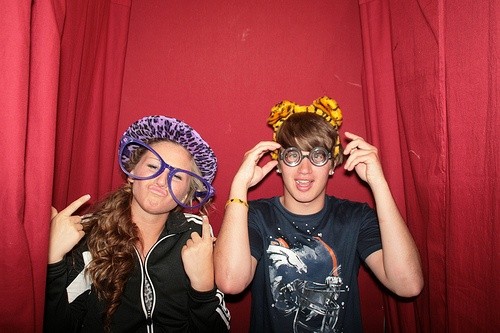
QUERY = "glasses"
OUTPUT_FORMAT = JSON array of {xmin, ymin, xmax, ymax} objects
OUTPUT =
[
  {"xmin": 119, "ymin": 137, "xmax": 211, "ymax": 210},
  {"xmin": 279, "ymin": 146, "xmax": 331, "ymax": 167}
]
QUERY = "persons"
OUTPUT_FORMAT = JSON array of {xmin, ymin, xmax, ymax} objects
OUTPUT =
[
  {"xmin": 44, "ymin": 114, "xmax": 231, "ymax": 332},
  {"xmin": 213, "ymin": 94, "xmax": 425, "ymax": 332}
]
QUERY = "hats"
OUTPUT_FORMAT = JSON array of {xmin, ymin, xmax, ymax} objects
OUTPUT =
[{"xmin": 118, "ymin": 116, "xmax": 217, "ymax": 195}]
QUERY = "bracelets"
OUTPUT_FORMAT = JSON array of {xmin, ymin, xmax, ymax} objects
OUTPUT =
[{"xmin": 225, "ymin": 197, "xmax": 249, "ymax": 212}]
